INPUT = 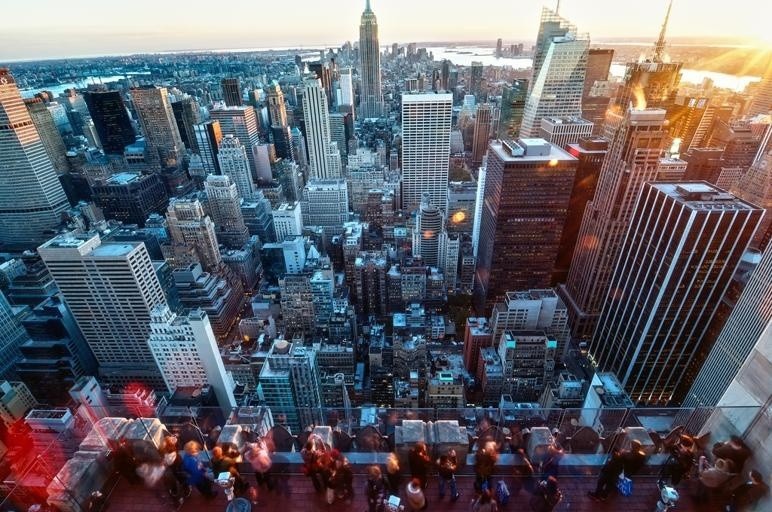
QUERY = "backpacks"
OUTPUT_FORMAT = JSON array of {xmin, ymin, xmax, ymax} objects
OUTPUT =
[
  {"xmin": 382, "ymin": 476, "xmax": 392, "ymax": 495},
  {"xmin": 157, "ymin": 473, "xmax": 182, "ymax": 504}
]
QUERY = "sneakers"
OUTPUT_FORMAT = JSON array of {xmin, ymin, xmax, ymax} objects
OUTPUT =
[
  {"xmin": 587, "ymin": 491, "xmax": 608, "ymax": 503},
  {"xmin": 316, "ymin": 487, "xmax": 354, "ymax": 503},
  {"xmin": 258, "ymin": 480, "xmax": 291, "ymax": 497},
  {"xmin": 204, "ymin": 491, "xmax": 219, "ymax": 498}
]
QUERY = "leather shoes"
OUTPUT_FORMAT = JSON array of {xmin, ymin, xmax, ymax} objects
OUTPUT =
[{"xmin": 440, "ymin": 493, "xmax": 459, "ymax": 501}]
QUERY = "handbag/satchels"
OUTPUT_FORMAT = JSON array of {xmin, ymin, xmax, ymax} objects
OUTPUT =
[
  {"xmin": 303, "ymin": 462, "xmax": 318, "ymax": 476},
  {"xmin": 495, "ymin": 480, "xmax": 510, "ymax": 506},
  {"xmin": 617, "ymin": 476, "xmax": 631, "ymax": 495},
  {"xmin": 326, "ymin": 488, "xmax": 335, "ymax": 503}
]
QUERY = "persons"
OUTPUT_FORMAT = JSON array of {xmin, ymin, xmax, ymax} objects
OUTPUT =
[
  {"xmin": 113, "ymin": 415, "xmax": 268, "ymax": 512},
  {"xmin": 299, "ymin": 434, "xmax": 355, "ymax": 506},
  {"xmin": 592, "ymin": 435, "xmax": 769, "ymax": 511},
  {"xmin": 365, "ymin": 440, "xmax": 569, "ymax": 512}
]
